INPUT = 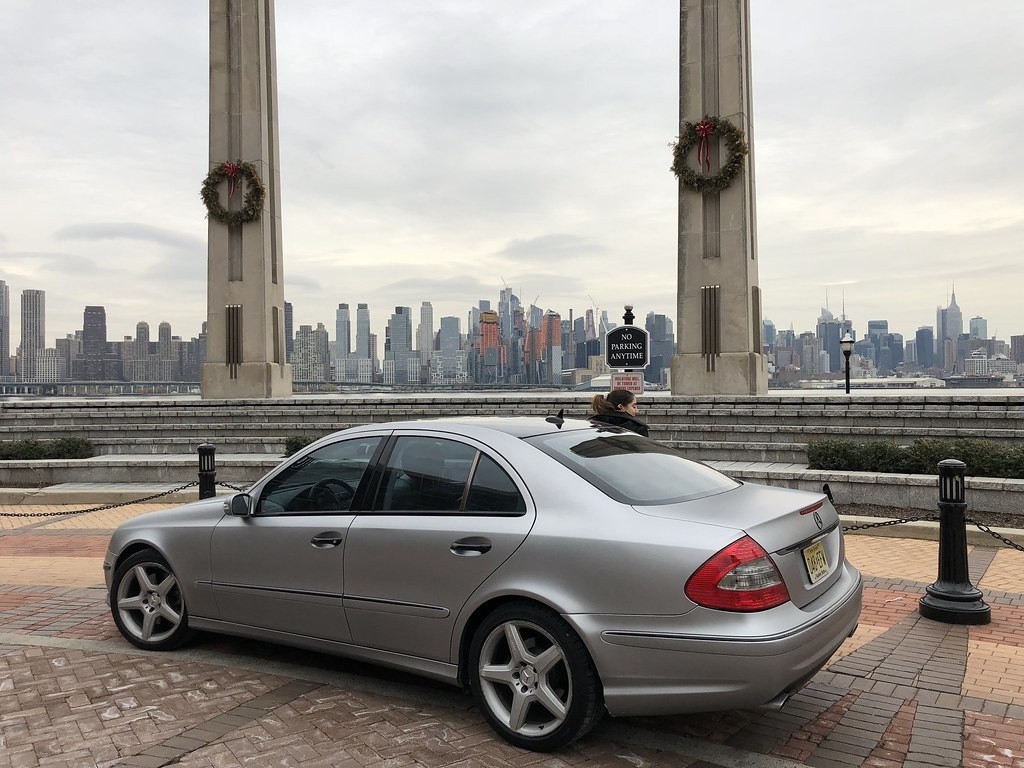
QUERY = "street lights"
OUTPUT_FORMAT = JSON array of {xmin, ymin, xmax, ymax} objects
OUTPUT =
[{"xmin": 839, "ymin": 330, "xmax": 855, "ymax": 394}]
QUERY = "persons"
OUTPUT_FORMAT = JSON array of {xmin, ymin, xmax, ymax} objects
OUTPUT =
[{"xmin": 588, "ymin": 388, "xmax": 649, "ymax": 435}]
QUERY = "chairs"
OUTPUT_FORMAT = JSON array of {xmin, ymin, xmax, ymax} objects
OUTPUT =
[{"xmin": 391, "ymin": 442, "xmax": 448, "ymax": 509}]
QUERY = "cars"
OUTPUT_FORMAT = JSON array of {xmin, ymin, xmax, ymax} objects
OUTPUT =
[{"xmin": 103, "ymin": 407, "xmax": 863, "ymax": 752}]
